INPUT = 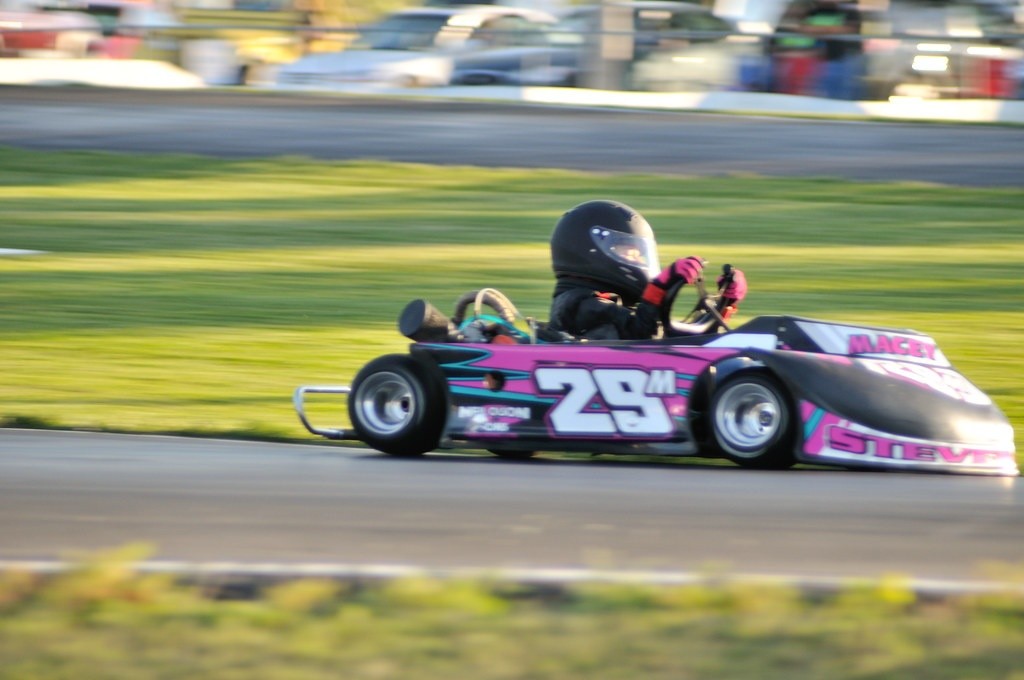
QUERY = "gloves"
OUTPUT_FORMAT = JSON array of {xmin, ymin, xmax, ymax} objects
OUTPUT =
[
  {"xmin": 653, "ymin": 256, "xmax": 704, "ymax": 294},
  {"xmin": 716, "ymin": 269, "xmax": 747, "ymax": 302}
]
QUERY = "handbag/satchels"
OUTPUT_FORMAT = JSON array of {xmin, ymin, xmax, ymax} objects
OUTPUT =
[{"xmin": 461, "ymin": 288, "xmax": 534, "ymax": 345}]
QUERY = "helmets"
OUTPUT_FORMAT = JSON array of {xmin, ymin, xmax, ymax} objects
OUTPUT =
[{"xmin": 550, "ymin": 200, "xmax": 661, "ymax": 296}]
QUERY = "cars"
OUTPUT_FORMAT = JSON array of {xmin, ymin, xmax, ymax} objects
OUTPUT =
[{"xmin": 1, "ymin": 0, "xmax": 1023, "ymax": 94}]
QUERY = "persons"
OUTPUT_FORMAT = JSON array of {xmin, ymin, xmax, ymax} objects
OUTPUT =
[
  {"xmin": 734, "ymin": 0, "xmax": 868, "ymax": 101},
  {"xmin": 547, "ymin": 200, "xmax": 748, "ymax": 341}
]
